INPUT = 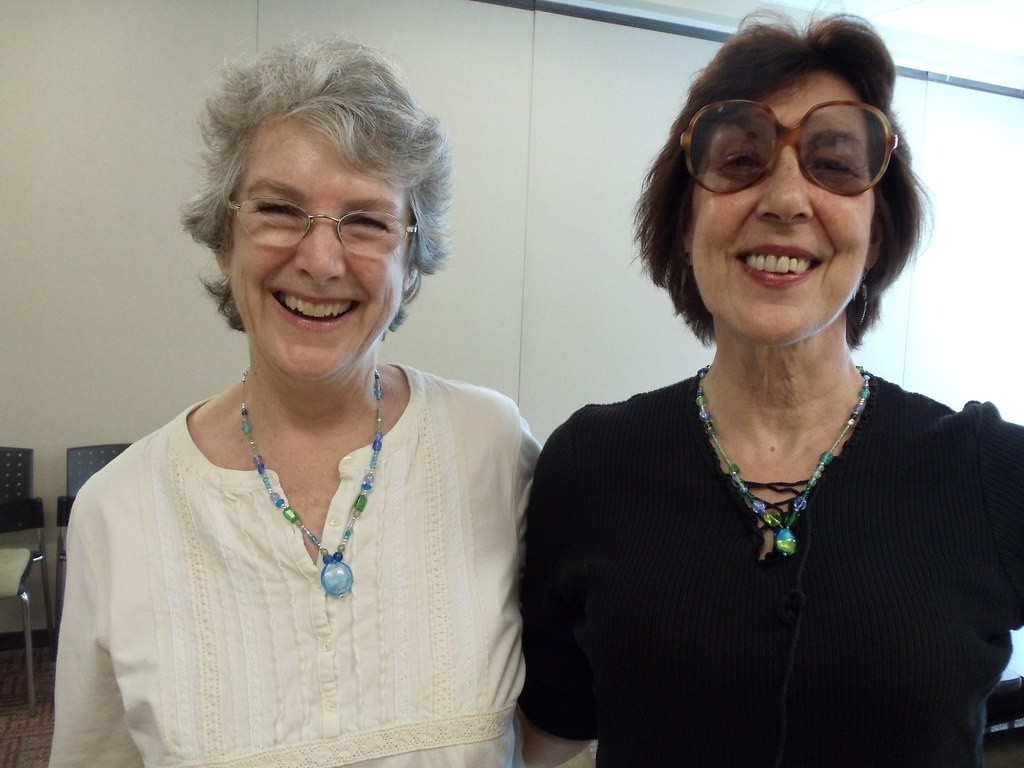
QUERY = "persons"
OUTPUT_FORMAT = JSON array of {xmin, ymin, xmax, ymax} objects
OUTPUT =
[
  {"xmin": 514, "ymin": 2, "xmax": 1023, "ymax": 768},
  {"xmin": 43, "ymin": 26, "xmax": 596, "ymax": 768}
]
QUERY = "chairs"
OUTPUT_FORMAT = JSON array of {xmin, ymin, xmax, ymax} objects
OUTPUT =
[
  {"xmin": 56, "ymin": 442, "xmax": 136, "ymax": 644},
  {"xmin": 0, "ymin": 445, "xmax": 57, "ymax": 662}
]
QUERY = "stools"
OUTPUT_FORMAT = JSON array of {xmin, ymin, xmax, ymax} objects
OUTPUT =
[{"xmin": 0, "ymin": 547, "xmax": 38, "ymax": 714}]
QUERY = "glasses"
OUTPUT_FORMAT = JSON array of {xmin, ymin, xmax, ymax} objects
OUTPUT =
[
  {"xmin": 225, "ymin": 196, "xmax": 419, "ymax": 256},
  {"xmin": 680, "ymin": 99, "xmax": 899, "ymax": 195}
]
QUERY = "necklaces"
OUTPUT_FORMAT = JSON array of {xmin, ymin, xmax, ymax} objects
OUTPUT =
[
  {"xmin": 694, "ymin": 363, "xmax": 872, "ymax": 558},
  {"xmin": 241, "ymin": 363, "xmax": 384, "ymax": 597}
]
